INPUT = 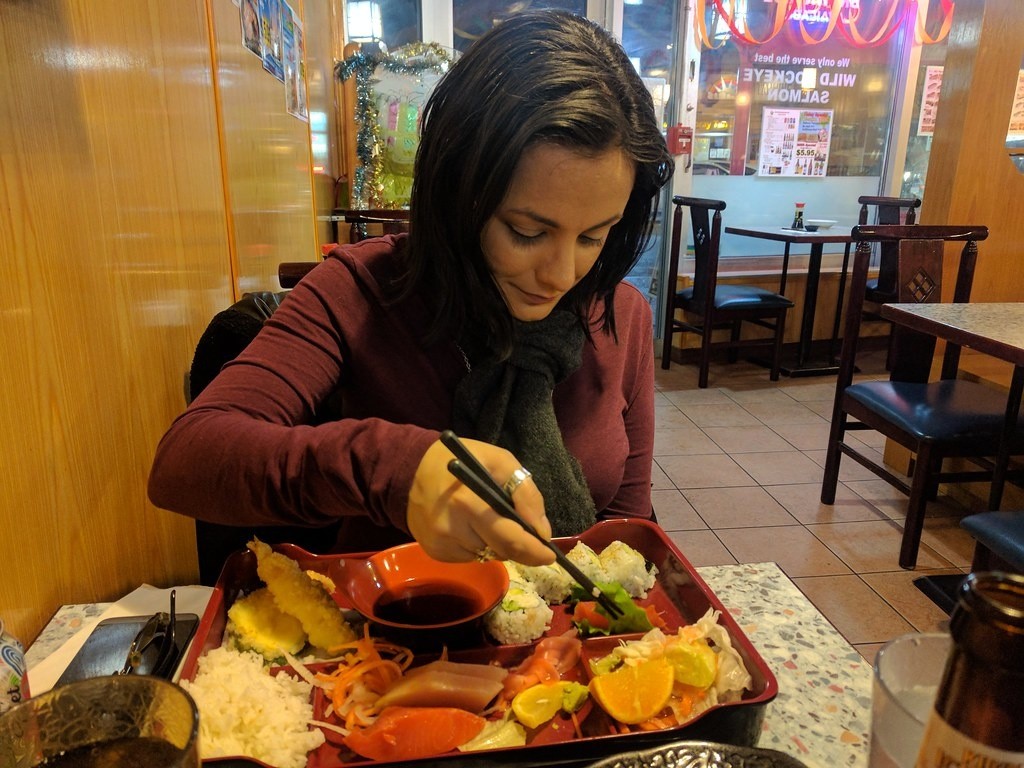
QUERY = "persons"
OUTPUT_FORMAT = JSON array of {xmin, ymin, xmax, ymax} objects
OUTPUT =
[{"xmin": 149, "ymin": 10, "xmax": 674, "ymax": 567}]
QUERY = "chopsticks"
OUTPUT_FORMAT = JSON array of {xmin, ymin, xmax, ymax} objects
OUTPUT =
[{"xmin": 439, "ymin": 429, "xmax": 626, "ymax": 621}]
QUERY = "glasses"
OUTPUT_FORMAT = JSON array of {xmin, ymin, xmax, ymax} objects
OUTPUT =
[{"xmin": 111, "ymin": 589, "xmax": 175, "ymax": 683}]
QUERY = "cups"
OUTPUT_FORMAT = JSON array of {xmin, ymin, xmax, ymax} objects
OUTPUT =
[
  {"xmin": 0, "ymin": 674, "xmax": 199, "ymax": 768},
  {"xmin": 867, "ymin": 633, "xmax": 955, "ymax": 768}
]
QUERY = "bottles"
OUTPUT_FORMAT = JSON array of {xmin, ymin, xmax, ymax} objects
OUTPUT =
[
  {"xmin": 808, "ymin": 159, "xmax": 812, "ymax": 175},
  {"xmin": 795, "ymin": 159, "xmax": 799, "ymax": 174},
  {"xmin": 819, "ymin": 160, "xmax": 823, "ymax": 175},
  {"xmin": 803, "ymin": 160, "xmax": 807, "ymax": 175},
  {"xmin": 814, "ymin": 162, "xmax": 818, "ymax": 175},
  {"xmin": 0, "ymin": 619, "xmax": 44, "ymax": 767},
  {"xmin": 914, "ymin": 569, "xmax": 1024, "ymax": 768},
  {"xmin": 791, "ymin": 202, "xmax": 805, "ymax": 229}
]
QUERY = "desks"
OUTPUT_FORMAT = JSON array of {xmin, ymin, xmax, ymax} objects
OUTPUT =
[
  {"xmin": 725, "ymin": 225, "xmax": 881, "ymax": 378},
  {"xmin": 25, "ymin": 559, "xmax": 873, "ymax": 768},
  {"xmin": 880, "ymin": 302, "xmax": 1024, "ymax": 570}
]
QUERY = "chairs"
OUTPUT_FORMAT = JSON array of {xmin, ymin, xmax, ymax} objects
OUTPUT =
[
  {"xmin": 188, "ymin": 290, "xmax": 336, "ymax": 587},
  {"xmin": 858, "ymin": 196, "xmax": 921, "ymax": 323},
  {"xmin": 660, "ymin": 196, "xmax": 795, "ymax": 390},
  {"xmin": 820, "ymin": 223, "xmax": 1024, "ymax": 569},
  {"xmin": 960, "ymin": 511, "xmax": 1024, "ymax": 573}
]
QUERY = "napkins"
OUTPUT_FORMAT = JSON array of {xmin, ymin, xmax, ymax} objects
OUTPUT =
[{"xmin": 26, "ymin": 581, "xmax": 244, "ymax": 697}]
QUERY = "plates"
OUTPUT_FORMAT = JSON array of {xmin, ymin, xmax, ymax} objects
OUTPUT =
[{"xmin": 153, "ymin": 518, "xmax": 778, "ymax": 768}]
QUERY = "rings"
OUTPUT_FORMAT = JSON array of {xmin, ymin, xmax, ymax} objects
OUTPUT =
[
  {"xmin": 475, "ymin": 546, "xmax": 495, "ymax": 563},
  {"xmin": 504, "ymin": 467, "xmax": 531, "ymax": 498}
]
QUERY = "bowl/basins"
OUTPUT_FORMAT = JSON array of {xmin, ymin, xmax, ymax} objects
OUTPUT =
[
  {"xmin": 805, "ymin": 226, "xmax": 820, "ymax": 231},
  {"xmin": 807, "ymin": 220, "xmax": 837, "ymax": 229},
  {"xmin": 326, "ymin": 541, "xmax": 512, "ymax": 644}
]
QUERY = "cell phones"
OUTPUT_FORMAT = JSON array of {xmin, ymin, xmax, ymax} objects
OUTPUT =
[{"xmin": 51, "ymin": 613, "xmax": 199, "ymax": 682}]
